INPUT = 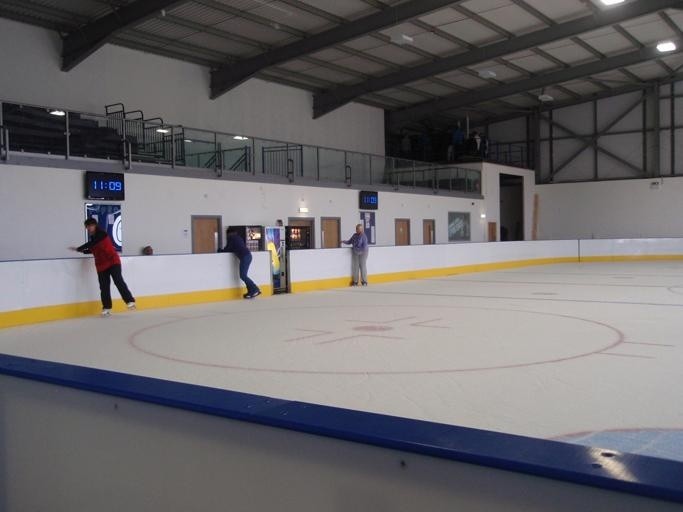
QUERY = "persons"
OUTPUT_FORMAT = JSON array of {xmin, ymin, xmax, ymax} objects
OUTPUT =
[
  {"xmin": 66, "ymin": 217, "xmax": 135, "ymax": 317},
  {"xmin": 142, "ymin": 246, "xmax": 152, "ymax": 255},
  {"xmin": 216, "ymin": 227, "xmax": 262, "ymax": 299},
  {"xmin": 340, "ymin": 223, "xmax": 368, "ymax": 288}
]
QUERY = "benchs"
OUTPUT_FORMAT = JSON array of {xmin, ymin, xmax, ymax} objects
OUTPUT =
[{"xmin": 2, "ymin": 102, "xmax": 138, "ymax": 160}]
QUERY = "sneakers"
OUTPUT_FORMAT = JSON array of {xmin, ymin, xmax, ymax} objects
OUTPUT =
[
  {"xmin": 244, "ymin": 290, "xmax": 260, "ymax": 298},
  {"xmin": 100, "ymin": 300, "xmax": 136, "ymax": 314},
  {"xmin": 350, "ymin": 281, "xmax": 367, "ymax": 287}
]
